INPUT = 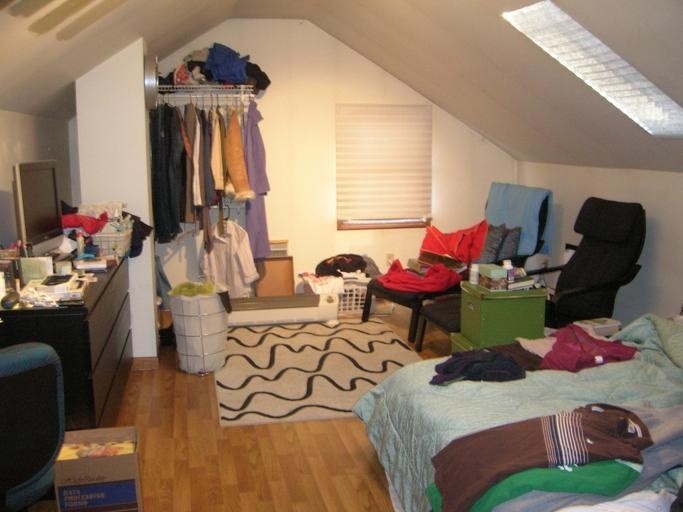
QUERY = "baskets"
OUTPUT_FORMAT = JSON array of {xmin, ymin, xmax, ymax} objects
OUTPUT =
[{"xmin": 338, "ymin": 284, "xmax": 377, "ymax": 314}]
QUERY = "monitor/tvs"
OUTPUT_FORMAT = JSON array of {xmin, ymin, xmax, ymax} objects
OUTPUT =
[{"xmin": 12, "ymin": 160, "xmax": 74, "ymax": 271}]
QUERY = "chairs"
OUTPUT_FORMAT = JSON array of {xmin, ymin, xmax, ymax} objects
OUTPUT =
[
  {"xmin": 414, "ymin": 193, "xmax": 646, "ymax": 352},
  {"xmin": 362, "ymin": 182, "xmax": 554, "ymax": 343},
  {"xmin": 0, "ymin": 342, "xmax": 67, "ymax": 508}
]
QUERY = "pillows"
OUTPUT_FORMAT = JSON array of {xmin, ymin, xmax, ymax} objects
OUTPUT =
[{"xmin": 421, "ymin": 220, "xmax": 523, "ymax": 265}]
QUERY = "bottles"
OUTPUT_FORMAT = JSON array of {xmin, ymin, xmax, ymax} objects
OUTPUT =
[
  {"xmin": 469, "ymin": 261, "xmax": 479, "ymax": 284},
  {"xmin": 502, "ymin": 259, "xmax": 514, "ymax": 282},
  {"xmin": 77, "ymin": 232, "xmax": 84, "ymax": 257}
]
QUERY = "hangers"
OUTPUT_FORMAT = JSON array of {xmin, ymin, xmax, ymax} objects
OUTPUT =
[
  {"xmin": 154, "ymin": 83, "xmax": 256, "ymax": 112},
  {"xmin": 222, "ymin": 202, "xmax": 232, "ymax": 222}
]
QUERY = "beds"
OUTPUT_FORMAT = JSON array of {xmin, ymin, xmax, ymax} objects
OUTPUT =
[{"xmin": 350, "ymin": 313, "xmax": 682, "ymax": 511}]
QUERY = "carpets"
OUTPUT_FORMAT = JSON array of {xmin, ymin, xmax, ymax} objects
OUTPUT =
[{"xmin": 213, "ymin": 314, "xmax": 423, "ymax": 428}]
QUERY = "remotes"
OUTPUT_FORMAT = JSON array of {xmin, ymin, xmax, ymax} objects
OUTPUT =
[{"xmin": 34, "ymin": 292, "xmax": 84, "ymax": 305}]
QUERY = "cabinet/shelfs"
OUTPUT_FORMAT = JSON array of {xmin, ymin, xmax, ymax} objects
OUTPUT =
[{"xmin": 0, "ymin": 255, "xmax": 133, "ymax": 430}]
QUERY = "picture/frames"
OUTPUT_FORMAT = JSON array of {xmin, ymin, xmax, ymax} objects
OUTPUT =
[{"xmin": 254, "ymin": 256, "xmax": 295, "ymax": 297}]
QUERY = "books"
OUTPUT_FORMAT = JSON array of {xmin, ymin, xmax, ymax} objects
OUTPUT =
[
  {"xmin": 574, "ymin": 317, "xmax": 621, "ymax": 337},
  {"xmin": 0, "ymin": 248, "xmax": 118, "ymax": 306},
  {"xmin": 506, "ymin": 276, "xmax": 534, "ymax": 290}
]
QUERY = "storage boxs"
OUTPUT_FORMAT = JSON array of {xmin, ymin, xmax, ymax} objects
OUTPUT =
[
  {"xmin": 51, "ymin": 425, "xmax": 143, "ymax": 511},
  {"xmin": 459, "ymin": 279, "xmax": 546, "ymax": 348},
  {"xmin": 449, "ymin": 332, "xmax": 475, "ymax": 354},
  {"xmin": 90, "ymin": 228, "xmax": 133, "ymax": 258}
]
